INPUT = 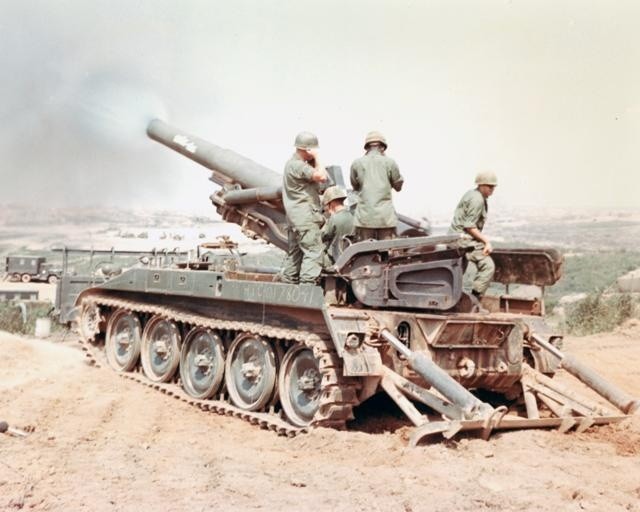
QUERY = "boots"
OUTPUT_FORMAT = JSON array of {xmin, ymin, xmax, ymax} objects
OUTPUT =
[{"xmin": 471, "ymin": 291, "xmax": 488, "ymax": 313}]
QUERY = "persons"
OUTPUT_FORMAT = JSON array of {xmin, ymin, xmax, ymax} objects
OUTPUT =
[
  {"xmin": 319, "ymin": 186, "xmax": 359, "ymax": 274},
  {"xmin": 350, "ymin": 132, "xmax": 404, "ymax": 240},
  {"xmin": 445, "ymin": 170, "xmax": 499, "ymax": 314},
  {"xmin": 273, "ymin": 130, "xmax": 329, "ymax": 286}
]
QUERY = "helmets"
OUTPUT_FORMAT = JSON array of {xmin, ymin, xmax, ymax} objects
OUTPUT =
[
  {"xmin": 475, "ymin": 170, "xmax": 498, "ymax": 187},
  {"xmin": 294, "ymin": 133, "xmax": 320, "ymax": 151},
  {"xmin": 364, "ymin": 132, "xmax": 387, "ymax": 151},
  {"xmin": 323, "ymin": 185, "xmax": 347, "ymax": 204}
]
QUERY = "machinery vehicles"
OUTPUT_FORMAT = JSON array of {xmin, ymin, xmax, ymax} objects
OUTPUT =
[{"xmin": 71, "ymin": 117, "xmax": 640, "ymax": 444}]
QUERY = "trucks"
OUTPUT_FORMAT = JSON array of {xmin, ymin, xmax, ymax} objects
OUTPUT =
[{"xmin": 5, "ymin": 255, "xmax": 63, "ymax": 284}]
